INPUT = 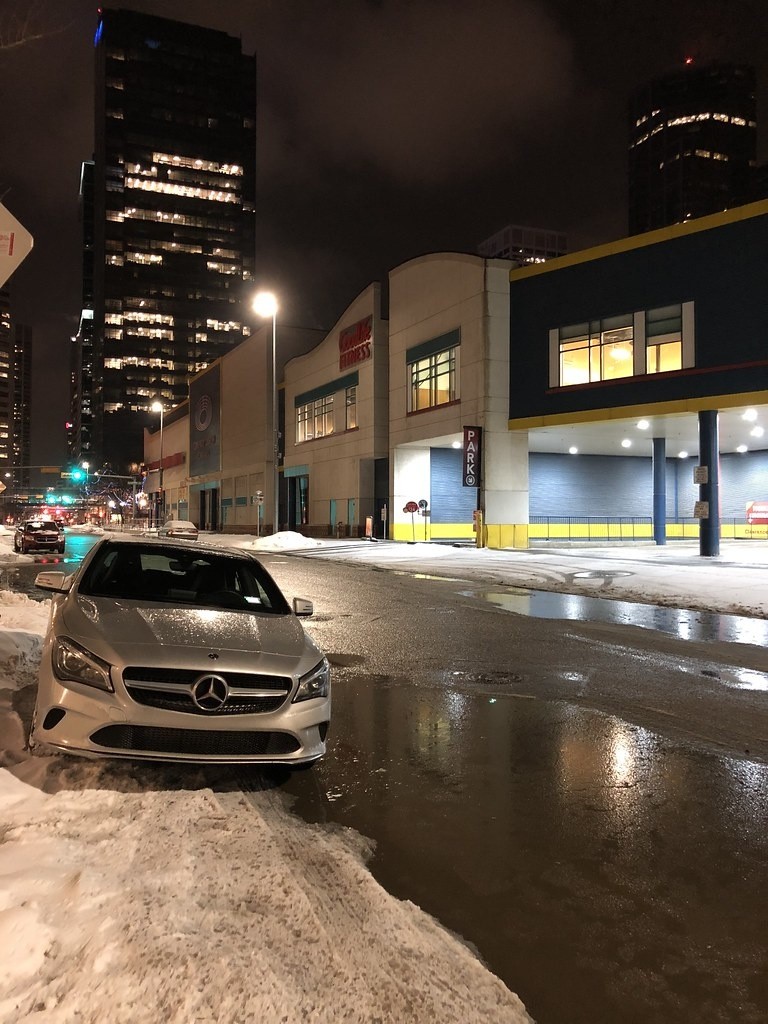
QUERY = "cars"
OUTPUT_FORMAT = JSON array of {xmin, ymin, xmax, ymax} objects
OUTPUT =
[
  {"xmin": 27, "ymin": 532, "xmax": 333, "ymax": 771},
  {"xmin": 156, "ymin": 519, "xmax": 200, "ymax": 543},
  {"xmin": 13, "ymin": 519, "xmax": 66, "ymax": 555}
]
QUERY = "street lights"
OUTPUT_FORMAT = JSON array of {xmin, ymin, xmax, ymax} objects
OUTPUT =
[
  {"xmin": 151, "ymin": 401, "xmax": 164, "ymax": 529},
  {"xmin": 82, "ymin": 462, "xmax": 89, "ymax": 481},
  {"xmin": 252, "ymin": 291, "xmax": 280, "ymax": 534}
]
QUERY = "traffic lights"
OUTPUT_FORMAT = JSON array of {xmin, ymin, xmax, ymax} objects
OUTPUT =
[{"xmin": 61, "ymin": 471, "xmax": 85, "ymax": 479}]
